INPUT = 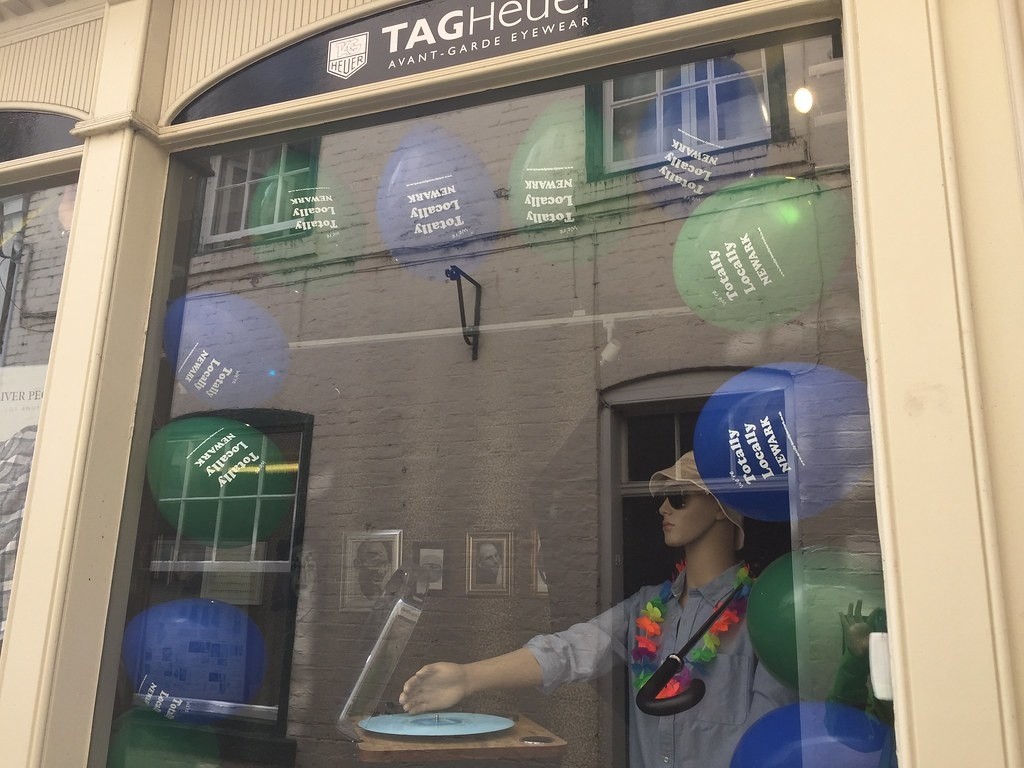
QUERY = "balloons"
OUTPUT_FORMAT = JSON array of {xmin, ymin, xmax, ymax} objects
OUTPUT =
[
  {"xmin": 746, "ymin": 543, "xmax": 889, "ymax": 706},
  {"xmin": 376, "ymin": 123, "xmax": 498, "ymax": 285},
  {"xmin": 730, "ymin": 698, "xmax": 898, "ymax": 768},
  {"xmin": 107, "ymin": 706, "xmax": 222, "ymax": 768},
  {"xmin": 692, "ymin": 362, "xmax": 870, "ymax": 524},
  {"xmin": 247, "ymin": 155, "xmax": 364, "ymax": 290},
  {"xmin": 508, "ymin": 95, "xmax": 635, "ymax": 264},
  {"xmin": 147, "ymin": 416, "xmax": 291, "ymax": 549},
  {"xmin": 636, "ymin": 59, "xmax": 770, "ymax": 209},
  {"xmin": 673, "ymin": 176, "xmax": 852, "ymax": 334},
  {"xmin": 121, "ymin": 599, "xmax": 266, "ymax": 725},
  {"xmin": 160, "ymin": 292, "xmax": 289, "ymax": 410}
]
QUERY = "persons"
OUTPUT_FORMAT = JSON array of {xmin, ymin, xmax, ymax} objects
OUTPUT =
[
  {"xmin": 824, "ymin": 602, "xmax": 901, "ymax": 768},
  {"xmin": 400, "ymin": 448, "xmax": 800, "ymax": 768},
  {"xmin": 476, "ymin": 543, "xmax": 501, "ymax": 584},
  {"xmin": 352, "ymin": 542, "xmax": 389, "ymax": 598}
]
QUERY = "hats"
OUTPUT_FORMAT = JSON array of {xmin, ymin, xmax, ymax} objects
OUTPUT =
[{"xmin": 645, "ymin": 446, "xmax": 746, "ymax": 552}]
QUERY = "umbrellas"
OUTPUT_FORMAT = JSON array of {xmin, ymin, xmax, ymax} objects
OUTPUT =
[{"xmin": 635, "ymin": 545, "xmax": 786, "ymax": 716}]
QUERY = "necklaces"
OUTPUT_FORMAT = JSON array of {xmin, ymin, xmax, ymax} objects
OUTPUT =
[{"xmin": 631, "ymin": 554, "xmax": 754, "ymax": 699}]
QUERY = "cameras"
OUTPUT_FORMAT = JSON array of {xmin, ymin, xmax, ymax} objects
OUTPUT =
[{"xmin": 872, "ymin": 609, "xmax": 887, "ymax": 632}]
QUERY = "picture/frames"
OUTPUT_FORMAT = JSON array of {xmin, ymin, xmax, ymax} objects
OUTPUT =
[
  {"xmin": 465, "ymin": 531, "xmax": 513, "ymax": 596},
  {"xmin": 531, "ymin": 527, "xmax": 548, "ymax": 596},
  {"xmin": 337, "ymin": 528, "xmax": 404, "ymax": 613},
  {"xmin": 413, "ymin": 540, "xmax": 449, "ymax": 597}
]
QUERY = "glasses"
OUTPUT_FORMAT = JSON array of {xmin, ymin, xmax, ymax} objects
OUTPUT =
[{"xmin": 652, "ymin": 486, "xmax": 707, "ymax": 508}]
